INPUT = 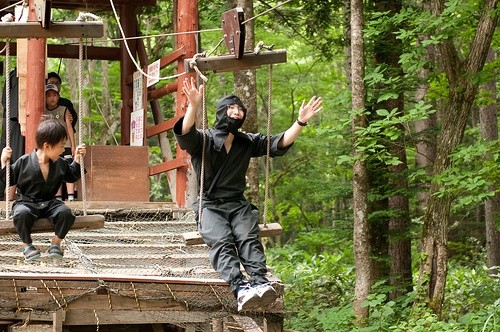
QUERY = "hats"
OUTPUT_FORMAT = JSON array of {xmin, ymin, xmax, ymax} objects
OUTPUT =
[{"xmin": 45, "ymin": 84, "xmax": 58, "ymax": 92}]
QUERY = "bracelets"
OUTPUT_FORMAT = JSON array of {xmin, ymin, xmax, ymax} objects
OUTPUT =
[{"xmin": 296, "ymin": 119, "xmax": 308, "ymax": 127}]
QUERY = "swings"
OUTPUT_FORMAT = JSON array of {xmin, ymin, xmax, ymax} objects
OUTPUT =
[
  {"xmin": 180, "ymin": 65, "xmax": 288, "ymax": 246},
  {"xmin": 0, "ymin": 36, "xmax": 106, "ymax": 235}
]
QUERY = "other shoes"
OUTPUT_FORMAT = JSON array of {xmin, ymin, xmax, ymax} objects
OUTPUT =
[
  {"xmin": 46, "ymin": 245, "xmax": 64, "ymax": 258},
  {"xmin": 23, "ymin": 245, "xmax": 41, "ymax": 261}
]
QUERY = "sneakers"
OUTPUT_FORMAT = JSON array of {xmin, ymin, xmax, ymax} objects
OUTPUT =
[
  {"xmin": 255, "ymin": 283, "xmax": 277, "ymax": 309},
  {"xmin": 237, "ymin": 287, "xmax": 259, "ymax": 312}
]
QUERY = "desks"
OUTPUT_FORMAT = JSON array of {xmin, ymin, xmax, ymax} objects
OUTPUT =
[{"xmin": 77, "ymin": 145, "xmax": 149, "ymax": 201}]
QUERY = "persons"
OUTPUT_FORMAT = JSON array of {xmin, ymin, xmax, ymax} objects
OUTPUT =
[
  {"xmin": 174, "ymin": 77, "xmax": 324, "ymax": 310},
  {"xmin": 0, "ymin": 66, "xmax": 88, "ymax": 261}
]
